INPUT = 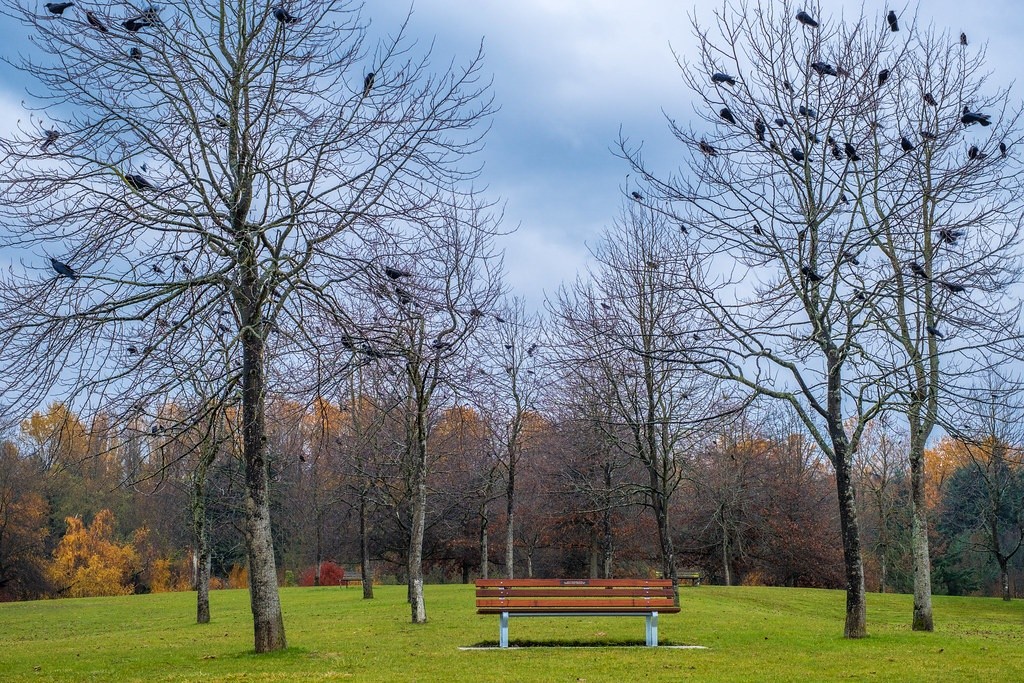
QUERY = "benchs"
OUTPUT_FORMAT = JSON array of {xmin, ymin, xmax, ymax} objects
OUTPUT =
[
  {"xmin": 474, "ymin": 577, "xmax": 681, "ymax": 646},
  {"xmin": 655, "ymin": 572, "xmax": 701, "ymax": 586},
  {"xmin": 341, "ymin": 571, "xmax": 374, "ymax": 586}
]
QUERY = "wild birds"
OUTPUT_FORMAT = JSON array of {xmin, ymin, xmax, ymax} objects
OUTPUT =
[{"xmin": 40, "ymin": 4, "xmax": 1010, "ymax": 469}]
